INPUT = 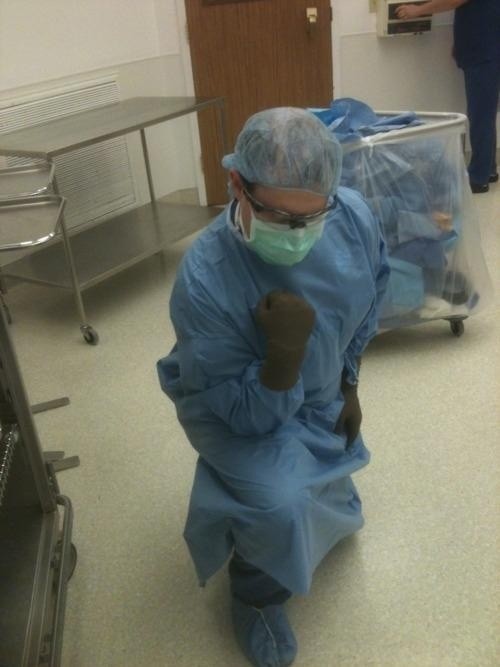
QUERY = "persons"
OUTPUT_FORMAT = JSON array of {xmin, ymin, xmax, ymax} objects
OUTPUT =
[
  {"xmin": 394, "ymin": 0, "xmax": 500, "ymax": 192},
  {"xmin": 156, "ymin": 108, "xmax": 389, "ymax": 667}
]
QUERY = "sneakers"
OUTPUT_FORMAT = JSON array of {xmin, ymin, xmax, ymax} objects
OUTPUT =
[
  {"xmin": 489, "ymin": 174, "xmax": 497, "ymax": 182},
  {"xmin": 472, "ymin": 184, "xmax": 488, "ymax": 193}
]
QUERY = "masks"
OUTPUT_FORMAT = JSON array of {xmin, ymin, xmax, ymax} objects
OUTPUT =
[{"xmin": 236, "ymin": 190, "xmax": 329, "ymax": 267}]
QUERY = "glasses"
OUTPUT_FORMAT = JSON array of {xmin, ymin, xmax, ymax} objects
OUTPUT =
[{"xmin": 240, "ymin": 182, "xmax": 339, "ymax": 228}]
